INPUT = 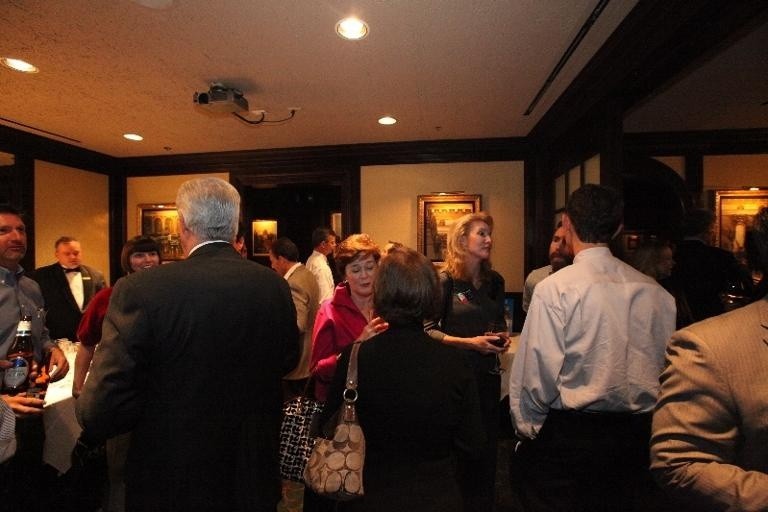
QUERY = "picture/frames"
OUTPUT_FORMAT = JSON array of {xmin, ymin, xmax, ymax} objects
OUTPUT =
[
  {"xmin": 710, "ymin": 186, "xmax": 768, "ymax": 284},
  {"xmin": 415, "ymin": 189, "xmax": 482, "ymax": 265},
  {"xmin": 251, "ymin": 220, "xmax": 281, "ymax": 257},
  {"xmin": 133, "ymin": 202, "xmax": 203, "ymax": 262}
]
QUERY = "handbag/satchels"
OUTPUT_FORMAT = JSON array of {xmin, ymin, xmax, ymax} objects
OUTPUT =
[
  {"xmin": 277, "ymin": 395, "xmax": 328, "ymax": 484},
  {"xmin": 303, "ymin": 404, "xmax": 365, "ymax": 500}
]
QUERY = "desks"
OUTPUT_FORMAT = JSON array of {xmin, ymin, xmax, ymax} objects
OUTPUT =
[{"xmin": 42, "ymin": 336, "xmax": 131, "ymax": 482}]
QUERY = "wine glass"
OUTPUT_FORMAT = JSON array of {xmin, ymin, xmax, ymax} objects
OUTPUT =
[{"xmin": 488, "ymin": 322, "xmax": 508, "ymax": 376}]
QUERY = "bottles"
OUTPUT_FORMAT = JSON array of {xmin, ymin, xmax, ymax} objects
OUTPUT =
[{"xmin": 1, "ymin": 313, "xmax": 34, "ymax": 397}]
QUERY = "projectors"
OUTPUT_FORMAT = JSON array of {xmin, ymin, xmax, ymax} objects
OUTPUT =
[{"xmin": 192, "ymin": 91, "xmax": 249, "ymax": 116}]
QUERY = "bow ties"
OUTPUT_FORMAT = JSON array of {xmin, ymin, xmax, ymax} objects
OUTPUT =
[{"xmin": 65, "ymin": 267, "xmax": 80, "ymax": 273}]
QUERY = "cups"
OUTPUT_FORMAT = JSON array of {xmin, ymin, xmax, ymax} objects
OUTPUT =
[
  {"xmin": 53, "ymin": 338, "xmax": 75, "ymax": 358},
  {"xmin": 719, "ymin": 278, "xmax": 747, "ymax": 313}
]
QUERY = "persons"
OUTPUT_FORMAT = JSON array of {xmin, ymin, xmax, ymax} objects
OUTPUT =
[{"xmin": 0, "ymin": 174, "xmax": 766, "ymax": 511}]
